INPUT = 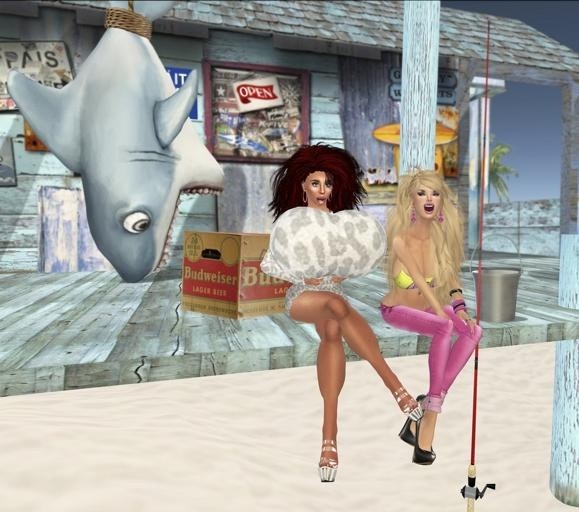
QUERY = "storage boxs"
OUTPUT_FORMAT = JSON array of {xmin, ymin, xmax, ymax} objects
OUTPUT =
[{"xmin": 180, "ymin": 230, "xmax": 288, "ymax": 321}]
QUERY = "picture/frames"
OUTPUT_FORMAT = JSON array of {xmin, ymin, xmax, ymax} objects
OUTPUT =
[{"xmin": 200, "ymin": 60, "xmax": 312, "ymax": 164}]
